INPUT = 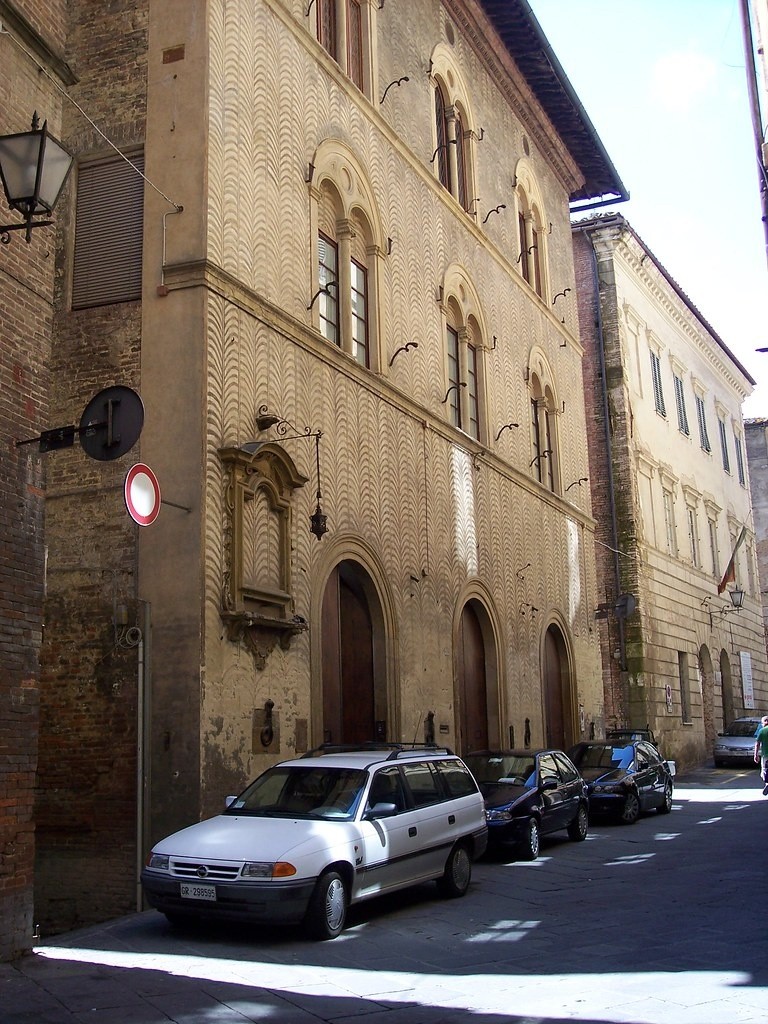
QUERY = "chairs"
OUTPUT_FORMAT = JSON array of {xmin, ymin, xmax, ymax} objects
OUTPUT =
[{"xmin": 281, "ymin": 784, "xmax": 319, "ymax": 819}]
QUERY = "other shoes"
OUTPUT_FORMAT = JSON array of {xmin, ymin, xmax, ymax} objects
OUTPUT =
[{"xmin": 763, "ymin": 785, "xmax": 768, "ymax": 795}]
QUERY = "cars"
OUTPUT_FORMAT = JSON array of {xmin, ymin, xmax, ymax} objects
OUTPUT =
[
  {"xmin": 462, "ymin": 747, "xmax": 588, "ymax": 862},
  {"xmin": 568, "ymin": 739, "xmax": 674, "ymax": 824}
]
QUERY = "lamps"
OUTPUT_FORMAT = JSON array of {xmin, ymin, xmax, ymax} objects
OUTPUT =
[
  {"xmin": 256, "ymin": 405, "xmax": 330, "ymax": 540},
  {"xmin": 709, "ymin": 585, "xmax": 746, "ymax": 633},
  {"xmin": 0, "ymin": 108, "xmax": 76, "ymax": 244}
]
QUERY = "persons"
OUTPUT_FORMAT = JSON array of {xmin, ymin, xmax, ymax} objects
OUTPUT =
[{"xmin": 753, "ymin": 716, "xmax": 768, "ymax": 796}]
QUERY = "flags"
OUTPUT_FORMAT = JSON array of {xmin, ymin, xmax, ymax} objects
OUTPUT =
[{"xmin": 718, "ymin": 527, "xmax": 747, "ymax": 595}]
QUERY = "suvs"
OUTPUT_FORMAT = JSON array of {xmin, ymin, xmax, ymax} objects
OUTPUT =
[
  {"xmin": 714, "ymin": 715, "xmax": 763, "ymax": 768},
  {"xmin": 139, "ymin": 741, "xmax": 488, "ymax": 940}
]
